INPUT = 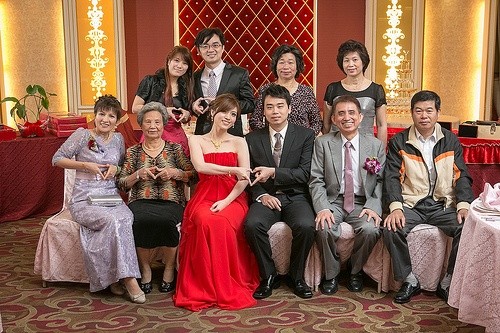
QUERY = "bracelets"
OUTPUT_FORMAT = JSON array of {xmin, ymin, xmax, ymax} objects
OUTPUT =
[
  {"xmin": 229, "ymin": 167, "xmax": 232, "ymax": 177},
  {"xmin": 176, "ymin": 169, "xmax": 185, "ymax": 180},
  {"xmin": 136, "ymin": 170, "xmax": 141, "ymax": 181},
  {"xmin": 173, "ymin": 167, "xmax": 179, "ymax": 181}
]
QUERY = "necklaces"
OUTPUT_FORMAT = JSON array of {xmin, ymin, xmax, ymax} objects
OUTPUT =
[
  {"xmin": 95, "ymin": 130, "xmax": 110, "ymax": 143},
  {"xmin": 209, "ymin": 132, "xmax": 228, "ymax": 150}
]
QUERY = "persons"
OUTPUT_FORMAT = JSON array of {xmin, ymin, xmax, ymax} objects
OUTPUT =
[
  {"xmin": 172, "ymin": 93, "xmax": 261, "ymax": 312},
  {"xmin": 384, "ymin": 90, "xmax": 473, "ymax": 304},
  {"xmin": 309, "ymin": 96, "xmax": 386, "ymax": 296},
  {"xmin": 248, "ymin": 45, "xmax": 324, "ymax": 138},
  {"xmin": 192, "ymin": 28, "xmax": 255, "ymax": 138},
  {"xmin": 118, "ymin": 102, "xmax": 198, "ymax": 294},
  {"xmin": 322, "ymin": 39, "xmax": 387, "ymax": 149},
  {"xmin": 51, "ymin": 94, "xmax": 146, "ymax": 303},
  {"xmin": 243, "ymin": 83, "xmax": 316, "ymax": 299},
  {"xmin": 132, "ymin": 46, "xmax": 192, "ymax": 160}
]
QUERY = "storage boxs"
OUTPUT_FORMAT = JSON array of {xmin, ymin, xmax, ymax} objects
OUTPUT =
[
  {"xmin": 40, "ymin": 111, "xmax": 88, "ymax": 137},
  {"xmin": 0, "ymin": 124, "xmax": 17, "ymax": 141}
]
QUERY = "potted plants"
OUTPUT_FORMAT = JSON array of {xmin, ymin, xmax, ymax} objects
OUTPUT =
[{"xmin": 0, "ymin": 84, "xmax": 57, "ymax": 138}]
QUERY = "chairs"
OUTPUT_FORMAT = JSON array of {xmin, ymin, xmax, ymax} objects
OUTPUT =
[
  {"xmin": 458, "ymin": 125, "xmax": 479, "ymax": 138},
  {"xmin": 35, "ymin": 155, "xmax": 453, "ymax": 293},
  {"xmin": 438, "ymin": 121, "xmax": 451, "ymax": 132}
]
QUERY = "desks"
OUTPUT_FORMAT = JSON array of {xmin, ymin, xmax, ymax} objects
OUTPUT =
[
  {"xmin": 373, "ymin": 126, "xmax": 500, "ymax": 199},
  {"xmin": 447, "ymin": 197, "xmax": 500, "ymax": 333},
  {"xmin": 0, "ymin": 131, "xmax": 69, "ymax": 223}
]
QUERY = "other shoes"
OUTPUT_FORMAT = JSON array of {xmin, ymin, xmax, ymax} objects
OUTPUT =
[
  {"xmin": 139, "ymin": 268, "xmax": 153, "ymax": 293},
  {"xmin": 159, "ymin": 268, "xmax": 176, "ymax": 292}
]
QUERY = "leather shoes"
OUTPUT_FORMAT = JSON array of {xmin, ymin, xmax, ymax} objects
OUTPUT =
[
  {"xmin": 437, "ymin": 281, "xmax": 451, "ymax": 304},
  {"xmin": 110, "ymin": 282, "xmax": 124, "ymax": 295},
  {"xmin": 252, "ymin": 273, "xmax": 281, "ymax": 299},
  {"xmin": 123, "ymin": 278, "xmax": 147, "ymax": 304},
  {"xmin": 346, "ymin": 258, "xmax": 364, "ymax": 292},
  {"xmin": 286, "ymin": 273, "xmax": 313, "ymax": 299},
  {"xmin": 320, "ymin": 274, "xmax": 338, "ymax": 295},
  {"xmin": 393, "ymin": 277, "xmax": 421, "ymax": 304}
]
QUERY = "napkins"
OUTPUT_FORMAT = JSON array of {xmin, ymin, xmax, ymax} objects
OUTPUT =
[{"xmin": 480, "ymin": 183, "xmax": 500, "ymax": 211}]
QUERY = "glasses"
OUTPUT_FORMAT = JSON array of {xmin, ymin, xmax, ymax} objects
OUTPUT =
[{"xmin": 199, "ymin": 42, "xmax": 222, "ymax": 49}]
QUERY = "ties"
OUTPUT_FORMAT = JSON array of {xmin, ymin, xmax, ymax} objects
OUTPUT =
[
  {"xmin": 208, "ymin": 71, "xmax": 218, "ymax": 104},
  {"xmin": 272, "ymin": 133, "xmax": 285, "ymax": 195},
  {"xmin": 342, "ymin": 142, "xmax": 356, "ymax": 215}
]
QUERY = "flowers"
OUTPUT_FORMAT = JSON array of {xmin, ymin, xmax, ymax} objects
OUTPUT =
[
  {"xmin": 89, "ymin": 139, "xmax": 103, "ymax": 153},
  {"xmin": 363, "ymin": 157, "xmax": 382, "ymax": 175}
]
